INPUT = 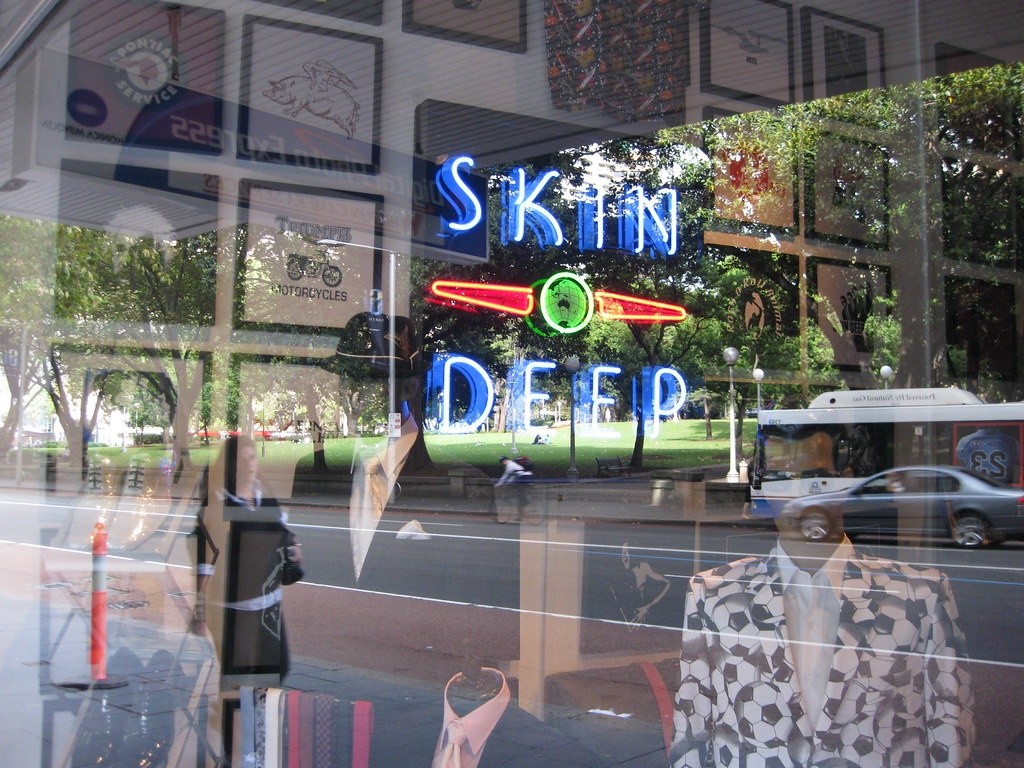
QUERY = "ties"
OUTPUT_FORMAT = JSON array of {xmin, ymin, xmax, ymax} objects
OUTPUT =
[
  {"xmin": 432, "ymin": 720, "xmax": 469, "ymax": 768},
  {"xmin": 352, "ymin": 449, "xmax": 383, "ymax": 583},
  {"xmin": 239, "ymin": 684, "xmax": 375, "ymax": 768}
]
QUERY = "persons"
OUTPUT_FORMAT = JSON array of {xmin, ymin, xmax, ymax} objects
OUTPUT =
[
  {"xmin": 670, "ymin": 424, "xmax": 977, "ymax": 768},
  {"xmin": 884, "ymin": 471, "xmax": 906, "ymax": 494},
  {"xmin": 532, "ymin": 433, "xmax": 551, "ymax": 447},
  {"xmin": 288, "ymin": 313, "xmax": 500, "ymax": 604},
  {"xmin": 490, "ymin": 457, "xmax": 532, "ymax": 521},
  {"xmin": 366, "ymin": 656, "xmax": 604, "ymax": 768},
  {"xmin": 740, "ymin": 443, "xmax": 762, "ymax": 520},
  {"xmin": 185, "ymin": 435, "xmax": 306, "ymax": 689}
]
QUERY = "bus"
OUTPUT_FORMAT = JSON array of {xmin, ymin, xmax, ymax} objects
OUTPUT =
[{"xmin": 742, "ymin": 387, "xmax": 1024, "ymax": 521}]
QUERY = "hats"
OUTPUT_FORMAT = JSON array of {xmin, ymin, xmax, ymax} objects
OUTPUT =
[
  {"xmin": 499, "ymin": 455, "xmax": 505, "ymax": 463},
  {"xmin": 321, "ymin": 312, "xmax": 434, "ymax": 378}
]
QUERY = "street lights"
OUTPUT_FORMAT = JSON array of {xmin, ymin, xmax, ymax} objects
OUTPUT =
[
  {"xmin": 565, "ymin": 356, "xmax": 580, "ymax": 479},
  {"xmin": 722, "ymin": 347, "xmax": 741, "ymax": 481},
  {"xmin": 880, "ymin": 365, "xmax": 893, "ymax": 389},
  {"xmin": 753, "ymin": 368, "xmax": 764, "ymax": 410},
  {"xmin": 318, "ymin": 239, "xmax": 395, "ymax": 504}
]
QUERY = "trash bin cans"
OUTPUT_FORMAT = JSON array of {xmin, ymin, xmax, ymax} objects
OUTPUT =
[
  {"xmin": 38, "ymin": 453, "xmax": 58, "ymax": 493},
  {"xmin": 651, "ymin": 480, "xmax": 674, "ymax": 506}
]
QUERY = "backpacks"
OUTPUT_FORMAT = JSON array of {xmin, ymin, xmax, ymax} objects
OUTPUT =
[{"xmin": 505, "ymin": 456, "xmax": 533, "ymax": 472}]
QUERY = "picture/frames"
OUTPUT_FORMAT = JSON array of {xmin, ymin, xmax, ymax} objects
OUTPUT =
[
  {"xmin": 402, "ymin": 0, "xmax": 528, "ymax": 54},
  {"xmin": 702, "ymin": 105, "xmax": 800, "ymax": 235},
  {"xmin": 580, "ymin": 520, "xmax": 694, "ymax": 655},
  {"xmin": 254, "ymin": 0, "xmax": 383, "ymax": 25},
  {"xmin": 806, "ymin": 255, "xmax": 892, "ymax": 373},
  {"xmin": 935, "ymin": 42, "xmax": 1009, "ymax": 155},
  {"xmin": 941, "ymin": 157, "xmax": 1013, "ymax": 268},
  {"xmin": 224, "ymin": 352, "xmax": 340, "ymax": 506},
  {"xmin": 699, "ymin": 0, "xmax": 794, "ymax": 112},
  {"xmin": 233, "ymin": 179, "xmax": 385, "ymax": 336},
  {"xmin": 55, "ymin": 159, "xmax": 220, "ymax": 325},
  {"xmin": 221, "ymin": 520, "xmax": 287, "ymax": 675},
  {"xmin": 704, "ymin": 244, "xmax": 801, "ymax": 368},
  {"xmin": 944, "ymin": 275, "xmax": 1016, "ymax": 383},
  {"xmin": 45, "ymin": 342, "xmax": 213, "ymax": 507},
  {"xmin": 64, "ymin": 0, "xmax": 224, "ymax": 156},
  {"xmin": 236, "ymin": 14, "xmax": 384, "ymax": 176},
  {"xmin": 800, "ymin": 6, "xmax": 887, "ymax": 130},
  {"xmin": 804, "ymin": 128, "xmax": 890, "ymax": 252}
]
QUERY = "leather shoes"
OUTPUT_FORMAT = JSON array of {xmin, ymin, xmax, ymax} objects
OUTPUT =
[
  {"xmin": 71, "ymin": 647, "xmax": 144, "ymax": 768},
  {"xmin": 107, "ymin": 449, "xmax": 184, "ymax": 552},
  {"xmin": 68, "ymin": 446, "xmax": 130, "ymax": 551},
  {"xmin": 111, "ymin": 649, "xmax": 186, "ymax": 768}
]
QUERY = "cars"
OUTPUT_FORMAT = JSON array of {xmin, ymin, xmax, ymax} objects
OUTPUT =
[
  {"xmin": 780, "ymin": 465, "xmax": 1024, "ymax": 549},
  {"xmin": 264, "ymin": 420, "xmax": 318, "ymax": 442}
]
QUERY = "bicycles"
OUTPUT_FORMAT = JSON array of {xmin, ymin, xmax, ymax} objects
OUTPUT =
[{"xmin": 490, "ymin": 482, "xmax": 544, "ymax": 525}]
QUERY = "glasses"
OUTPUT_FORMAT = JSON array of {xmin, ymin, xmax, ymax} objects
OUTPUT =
[{"xmin": 761, "ymin": 468, "xmax": 845, "ymax": 483}]
